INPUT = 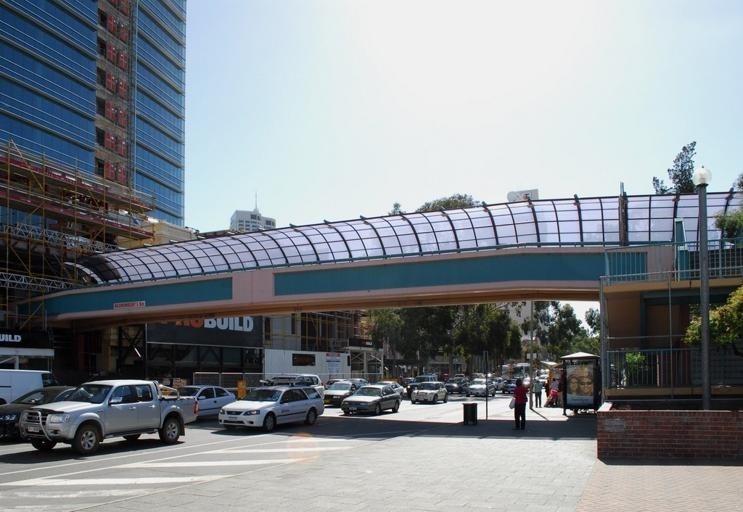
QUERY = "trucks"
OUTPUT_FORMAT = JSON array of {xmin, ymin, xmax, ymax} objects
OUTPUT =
[{"xmin": 0, "ymin": 369, "xmax": 57, "ymax": 410}]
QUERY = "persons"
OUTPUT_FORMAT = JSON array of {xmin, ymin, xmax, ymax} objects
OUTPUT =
[
  {"xmin": 549, "ymin": 378, "xmax": 559, "ymax": 407},
  {"xmin": 533, "ymin": 381, "xmax": 542, "ymax": 408},
  {"xmin": 513, "ymin": 379, "xmax": 529, "ymax": 430},
  {"xmin": 545, "ymin": 380, "xmax": 549, "ymax": 398},
  {"xmin": 568, "ymin": 371, "xmax": 593, "ymax": 396}
]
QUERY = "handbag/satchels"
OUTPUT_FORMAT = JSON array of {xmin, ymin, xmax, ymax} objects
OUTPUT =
[{"xmin": 508, "ymin": 397, "xmax": 516, "ymax": 410}]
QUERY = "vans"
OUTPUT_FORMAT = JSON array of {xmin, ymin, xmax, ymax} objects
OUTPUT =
[{"xmin": 258, "ymin": 373, "xmax": 325, "ymax": 389}]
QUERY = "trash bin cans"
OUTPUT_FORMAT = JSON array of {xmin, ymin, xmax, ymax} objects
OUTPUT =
[{"xmin": 464, "ymin": 403, "xmax": 477, "ymax": 425}]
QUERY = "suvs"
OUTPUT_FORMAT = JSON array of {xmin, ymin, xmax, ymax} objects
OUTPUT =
[{"xmin": 406, "ymin": 375, "xmax": 437, "ymax": 398}]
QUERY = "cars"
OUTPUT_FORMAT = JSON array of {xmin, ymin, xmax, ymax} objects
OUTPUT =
[
  {"xmin": 322, "ymin": 378, "xmax": 346, "ymax": 390},
  {"xmin": 401, "ymin": 377, "xmax": 414, "ymax": 385},
  {"xmin": 339, "ymin": 385, "xmax": 400, "ymax": 414},
  {"xmin": 373, "ymin": 379, "xmax": 404, "ymax": 399},
  {"xmin": 321, "ymin": 380, "xmax": 365, "ymax": 406},
  {"xmin": 169, "ymin": 383, "xmax": 235, "ymax": 418},
  {"xmin": 0, "ymin": 385, "xmax": 77, "ymax": 440},
  {"xmin": 410, "ymin": 381, "xmax": 448, "ymax": 404},
  {"xmin": 348, "ymin": 378, "xmax": 369, "ymax": 388},
  {"xmin": 216, "ymin": 384, "xmax": 324, "ymax": 432},
  {"xmin": 445, "ymin": 359, "xmax": 554, "ymax": 399}
]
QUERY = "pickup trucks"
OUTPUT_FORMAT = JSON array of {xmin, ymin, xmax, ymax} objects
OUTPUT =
[{"xmin": 19, "ymin": 378, "xmax": 200, "ymax": 454}]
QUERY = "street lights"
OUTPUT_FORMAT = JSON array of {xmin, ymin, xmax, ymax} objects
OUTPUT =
[{"xmin": 692, "ymin": 164, "xmax": 713, "ymax": 410}]
